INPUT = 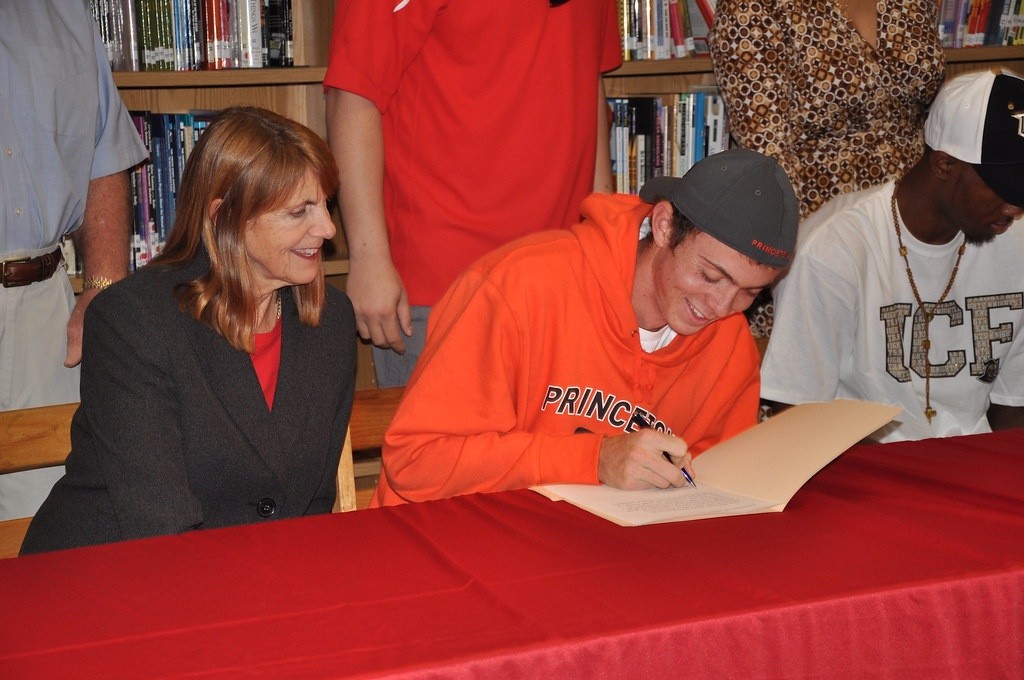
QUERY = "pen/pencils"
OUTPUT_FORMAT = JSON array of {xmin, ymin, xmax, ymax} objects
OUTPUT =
[{"xmin": 631, "ymin": 413, "xmax": 697, "ymax": 488}]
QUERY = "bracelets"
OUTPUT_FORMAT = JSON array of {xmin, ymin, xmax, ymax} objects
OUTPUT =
[{"xmin": 82, "ymin": 277, "xmax": 113, "ymax": 290}]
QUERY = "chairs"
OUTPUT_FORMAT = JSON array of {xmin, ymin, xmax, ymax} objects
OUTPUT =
[{"xmin": 0, "ymin": 404, "xmax": 356, "ymax": 562}]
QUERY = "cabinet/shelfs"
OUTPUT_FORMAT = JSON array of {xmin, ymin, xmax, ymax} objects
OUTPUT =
[{"xmin": 83, "ymin": 1, "xmax": 1024, "ymax": 387}]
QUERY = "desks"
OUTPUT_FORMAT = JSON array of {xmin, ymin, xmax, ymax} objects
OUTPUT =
[{"xmin": 0, "ymin": 428, "xmax": 1023, "ymax": 680}]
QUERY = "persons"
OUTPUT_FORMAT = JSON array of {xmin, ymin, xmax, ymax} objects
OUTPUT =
[
  {"xmin": 0, "ymin": 0, "xmax": 149, "ymax": 521},
  {"xmin": 367, "ymin": 148, "xmax": 799, "ymax": 508},
  {"xmin": 322, "ymin": 0, "xmax": 623, "ymax": 387},
  {"xmin": 760, "ymin": 67, "xmax": 1024, "ymax": 444},
  {"xmin": 709, "ymin": 1, "xmax": 946, "ymax": 423},
  {"xmin": 17, "ymin": 106, "xmax": 358, "ymax": 556}
]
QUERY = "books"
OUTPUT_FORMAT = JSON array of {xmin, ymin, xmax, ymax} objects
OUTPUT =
[
  {"xmin": 939, "ymin": 0, "xmax": 1024, "ymax": 47},
  {"xmin": 90, "ymin": 0, "xmax": 294, "ymax": 71},
  {"xmin": 619, "ymin": 0, "xmax": 718, "ymax": 60},
  {"xmin": 607, "ymin": 94, "xmax": 737, "ymax": 195},
  {"xmin": 61, "ymin": 110, "xmax": 211, "ymax": 277},
  {"xmin": 531, "ymin": 400, "xmax": 904, "ymax": 526}
]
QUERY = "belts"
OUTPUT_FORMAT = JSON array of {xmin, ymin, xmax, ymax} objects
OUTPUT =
[{"xmin": 0, "ymin": 246, "xmax": 61, "ymax": 288}]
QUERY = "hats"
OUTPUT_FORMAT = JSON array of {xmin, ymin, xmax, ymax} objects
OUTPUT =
[
  {"xmin": 924, "ymin": 66, "xmax": 1024, "ymax": 207},
  {"xmin": 640, "ymin": 148, "xmax": 799, "ymax": 268}
]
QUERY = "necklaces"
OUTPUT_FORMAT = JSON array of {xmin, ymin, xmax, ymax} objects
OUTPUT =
[{"xmin": 892, "ymin": 180, "xmax": 966, "ymax": 425}]
